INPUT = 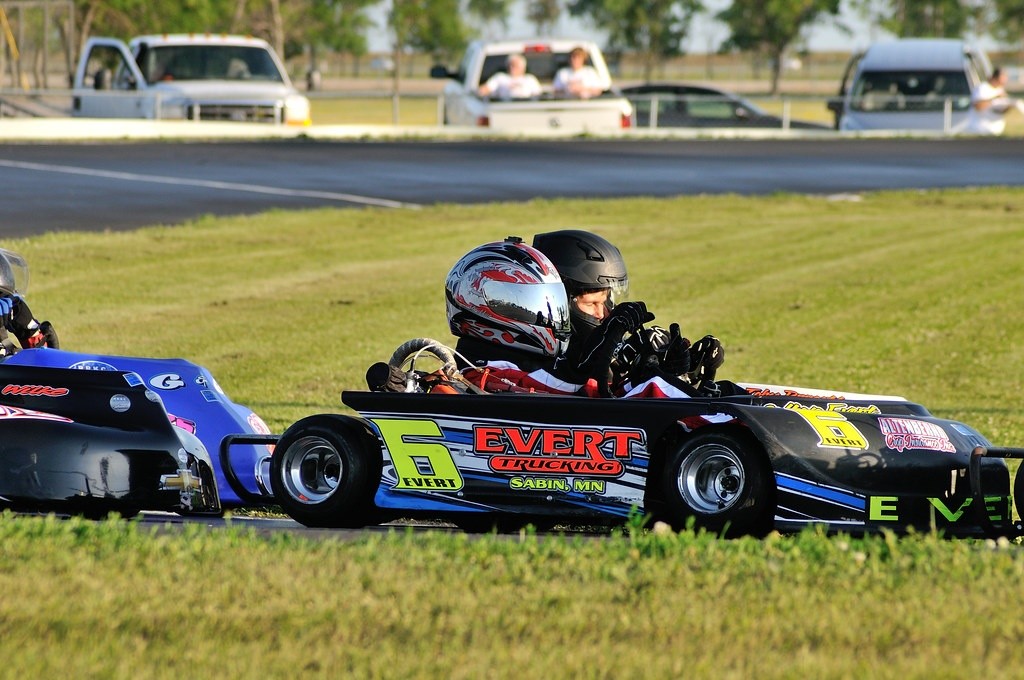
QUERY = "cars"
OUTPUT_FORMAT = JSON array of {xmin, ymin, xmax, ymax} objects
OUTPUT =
[{"xmin": 611, "ymin": 80, "xmax": 837, "ymax": 133}]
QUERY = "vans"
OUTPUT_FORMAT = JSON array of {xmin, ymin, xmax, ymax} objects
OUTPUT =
[{"xmin": 825, "ymin": 37, "xmax": 1003, "ymax": 135}]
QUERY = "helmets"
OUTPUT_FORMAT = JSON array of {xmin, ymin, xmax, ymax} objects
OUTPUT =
[
  {"xmin": 444, "ymin": 236, "xmax": 571, "ymax": 358},
  {"xmin": 0, "ymin": 248, "xmax": 29, "ymax": 298},
  {"xmin": 532, "ymin": 230, "xmax": 629, "ymax": 339}
]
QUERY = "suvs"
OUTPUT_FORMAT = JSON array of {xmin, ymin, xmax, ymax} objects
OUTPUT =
[{"xmin": 69, "ymin": 30, "xmax": 314, "ymax": 131}]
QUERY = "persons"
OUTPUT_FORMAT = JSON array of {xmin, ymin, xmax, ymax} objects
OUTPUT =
[
  {"xmin": 447, "ymin": 229, "xmax": 723, "ymax": 400},
  {"xmin": 0, "ymin": 249, "xmax": 46, "ymax": 356},
  {"xmin": 970, "ymin": 67, "xmax": 1014, "ymax": 138},
  {"xmin": 472, "ymin": 47, "xmax": 608, "ymax": 102}
]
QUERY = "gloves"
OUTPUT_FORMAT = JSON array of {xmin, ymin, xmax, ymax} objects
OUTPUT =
[
  {"xmin": 690, "ymin": 335, "xmax": 725, "ymax": 370},
  {"xmin": 618, "ymin": 326, "xmax": 670, "ymax": 366},
  {"xmin": 658, "ymin": 324, "xmax": 691, "ymax": 377},
  {"xmin": 597, "ymin": 302, "xmax": 655, "ymax": 343}
]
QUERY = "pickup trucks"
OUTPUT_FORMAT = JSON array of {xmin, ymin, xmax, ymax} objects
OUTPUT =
[{"xmin": 431, "ymin": 37, "xmax": 638, "ymax": 131}]
